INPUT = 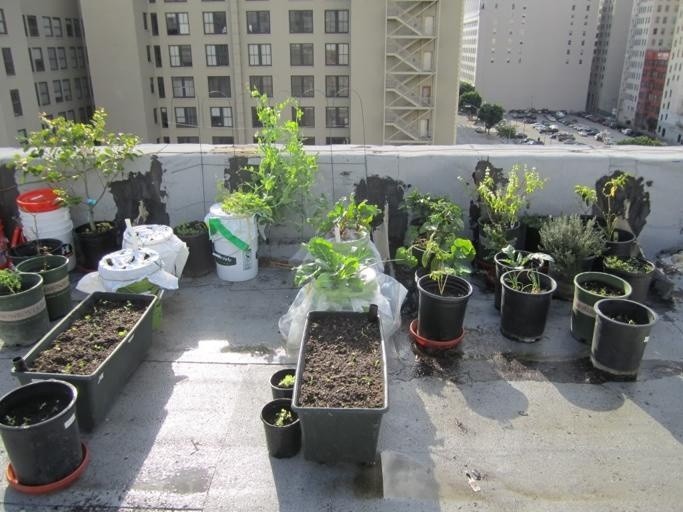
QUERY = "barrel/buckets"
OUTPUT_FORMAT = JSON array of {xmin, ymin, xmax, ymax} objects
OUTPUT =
[
  {"xmin": 122, "ymin": 223, "xmax": 189, "ymax": 278},
  {"xmin": 95, "ymin": 249, "xmax": 167, "ymax": 333},
  {"xmin": 209, "ymin": 202, "xmax": 258, "ymax": 282},
  {"xmin": 18, "ymin": 208, "xmax": 77, "ymax": 271},
  {"xmin": 570, "ymin": 271, "xmax": 631, "ymax": 344}
]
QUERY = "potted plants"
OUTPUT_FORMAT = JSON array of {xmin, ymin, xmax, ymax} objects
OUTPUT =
[
  {"xmin": 173, "ymin": 220, "xmax": 216, "ymax": 278},
  {"xmin": 260, "ymin": 400, "xmax": 302, "ymax": 458},
  {"xmin": 269, "ymin": 368, "xmax": 296, "ymax": 399},
  {"xmin": 11, "ymin": 292, "xmax": 158, "ymax": 433},
  {"xmin": 304, "ymin": 191, "xmax": 382, "ymax": 255},
  {"xmin": 209, "ymin": 85, "xmax": 320, "ymax": 281},
  {"xmin": 294, "ymin": 235, "xmax": 380, "ymax": 310},
  {"xmin": 291, "ymin": 311, "xmax": 390, "ymax": 463},
  {"xmin": 6, "ymin": 105, "xmax": 145, "ymax": 276}
]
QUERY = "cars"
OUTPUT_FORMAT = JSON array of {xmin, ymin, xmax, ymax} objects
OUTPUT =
[{"xmin": 461, "ymin": 105, "xmax": 636, "ymax": 145}]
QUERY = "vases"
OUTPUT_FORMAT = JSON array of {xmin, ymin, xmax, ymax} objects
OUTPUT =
[{"xmin": 5, "ymin": 238, "xmax": 63, "ymax": 267}]
